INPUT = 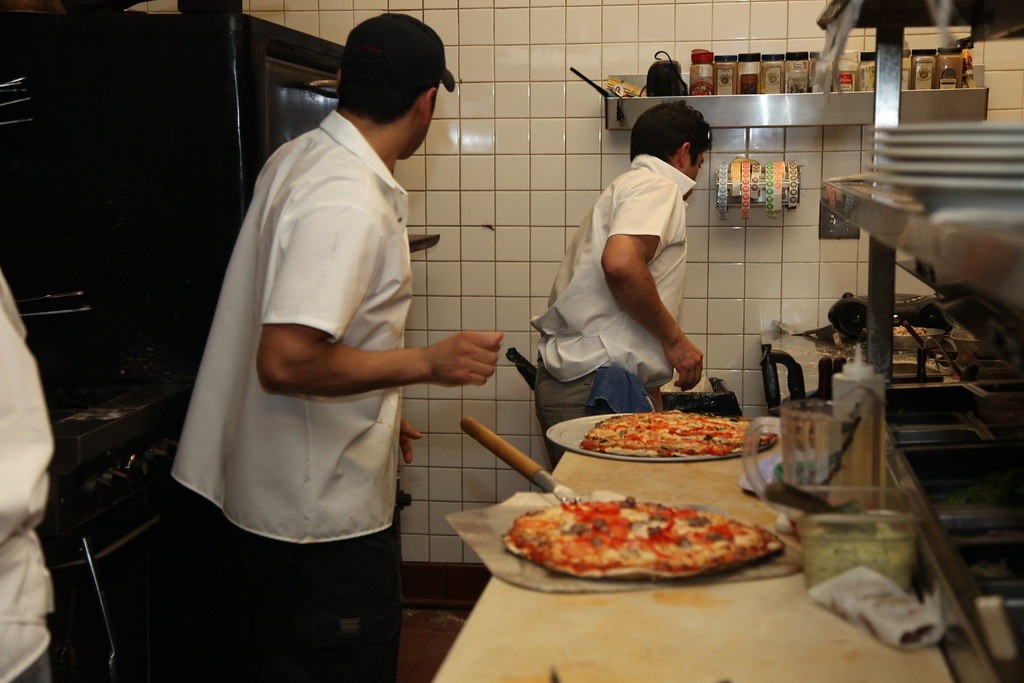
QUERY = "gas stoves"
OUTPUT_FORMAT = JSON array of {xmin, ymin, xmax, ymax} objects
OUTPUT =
[{"xmin": 40, "ymin": 380, "xmax": 190, "ymax": 514}]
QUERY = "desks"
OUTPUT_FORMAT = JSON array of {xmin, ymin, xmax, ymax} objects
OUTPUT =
[{"xmin": 430, "ymin": 421, "xmax": 961, "ymax": 683}]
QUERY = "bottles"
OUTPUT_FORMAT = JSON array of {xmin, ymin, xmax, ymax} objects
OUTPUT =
[
  {"xmin": 689, "ymin": 49, "xmax": 876, "ymax": 96},
  {"xmin": 901, "ymin": 48, "xmax": 976, "ymax": 91},
  {"xmin": 832, "ymin": 343, "xmax": 887, "ymax": 511}
]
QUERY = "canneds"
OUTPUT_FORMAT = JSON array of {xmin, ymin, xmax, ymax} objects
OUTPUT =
[{"xmin": 688, "ymin": 47, "xmax": 963, "ymax": 96}]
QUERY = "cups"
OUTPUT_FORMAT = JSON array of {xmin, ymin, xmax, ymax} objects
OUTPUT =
[{"xmin": 742, "ymin": 399, "xmax": 833, "ymax": 498}]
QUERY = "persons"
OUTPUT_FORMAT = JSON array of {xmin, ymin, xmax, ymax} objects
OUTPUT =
[
  {"xmin": 529, "ymin": 99, "xmax": 713, "ymax": 471},
  {"xmin": 170, "ymin": 12, "xmax": 504, "ymax": 683}
]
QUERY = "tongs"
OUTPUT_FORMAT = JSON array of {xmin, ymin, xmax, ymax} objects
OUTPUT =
[
  {"xmin": 55, "ymin": 535, "xmax": 124, "ymax": 683},
  {"xmin": 921, "ymin": 336, "xmax": 967, "ymax": 383}
]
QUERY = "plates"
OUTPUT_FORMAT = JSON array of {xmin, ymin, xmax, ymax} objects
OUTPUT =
[
  {"xmin": 861, "ymin": 120, "xmax": 1024, "ymax": 215},
  {"xmin": 545, "ymin": 412, "xmax": 777, "ymax": 462}
]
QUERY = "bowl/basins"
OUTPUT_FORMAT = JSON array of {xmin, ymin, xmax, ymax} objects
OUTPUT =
[
  {"xmin": 796, "ymin": 483, "xmax": 922, "ymax": 594},
  {"xmin": 894, "ymin": 326, "xmax": 946, "ymax": 350},
  {"xmin": 950, "ymin": 330, "xmax": 987, "ymax": 353}
]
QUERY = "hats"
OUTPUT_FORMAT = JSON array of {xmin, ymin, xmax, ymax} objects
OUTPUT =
[{"xmin": 346, "ymin": 13, "xmax": 456, "ymax": 93}]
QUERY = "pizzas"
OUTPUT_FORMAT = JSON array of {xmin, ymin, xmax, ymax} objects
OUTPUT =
[
  {"xmin": 579, "ymin": 410, "xmax": 777, "ymax": 457},
  {"xmin": 499, "ymin": 496, "xmax": 784, "ymax": 578}
]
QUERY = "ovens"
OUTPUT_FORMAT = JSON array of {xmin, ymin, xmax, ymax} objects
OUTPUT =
[
  {"xmin": 2, "ymin": 11, "xmax": 440, "ymax": 372},
  {"xmin": 51, "ymin": 479, "xmax": 208, "ymax": 683}
]
